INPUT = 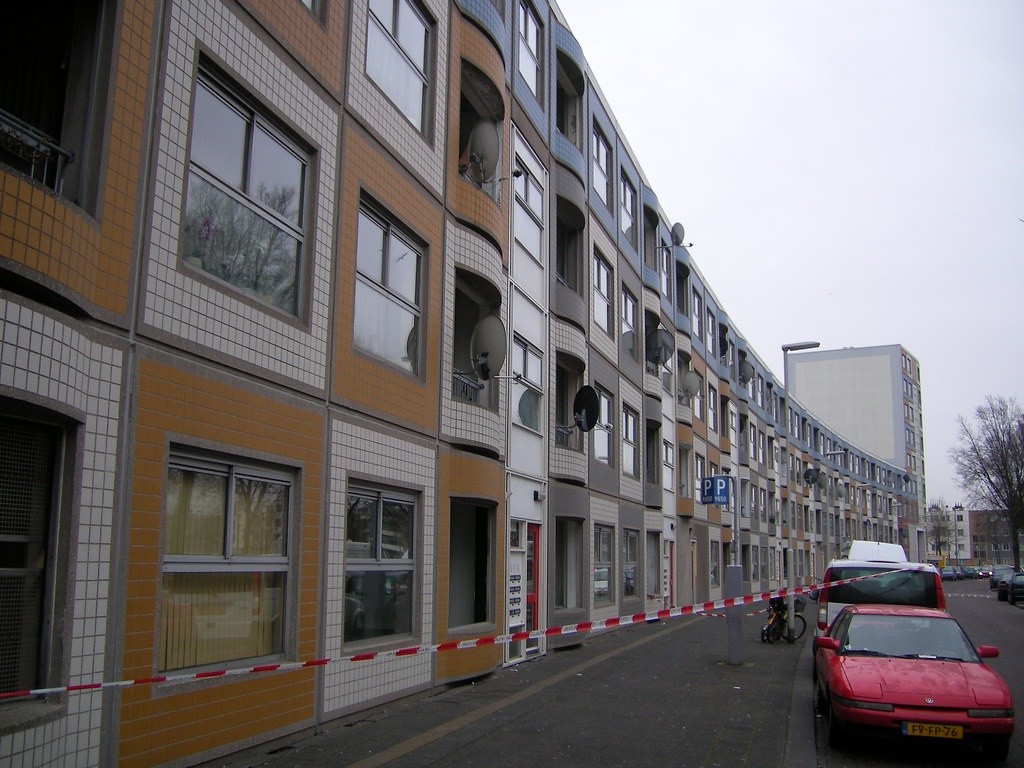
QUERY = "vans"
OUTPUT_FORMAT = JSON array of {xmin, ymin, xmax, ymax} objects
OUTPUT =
[{"xmin": 807, "ymin": 540, "xmax": 947, "ymax": 653}]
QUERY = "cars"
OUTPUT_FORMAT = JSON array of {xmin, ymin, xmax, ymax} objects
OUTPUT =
[
  {"xmin": 936, "ymin": 564, "xmax": 991, "ymax": 581},
  {"xmin": 808, "ymin": 607, "xmax": 1016, "ymax": 764},
  {"xmin": 996, "ymin": 574, "xmax": 1013, "ymax": 601}
]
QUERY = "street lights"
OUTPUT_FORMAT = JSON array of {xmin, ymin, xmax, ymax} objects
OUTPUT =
[
  {"xmin": 952, "ymin": 505, "xmax": 960, "ymax": 567},
  {"xmin": 921, "ymin": 515, "xmax": 932, "ymax": 563},
  {"xmin": 824, "ymin": 450, "xmax": 846, "ymax": 569},
  {"xmin": 858, "ymin": 483, "xmax": 871, "ymax": 540},
  {"xmin": 781, "ymin": 340, "xmax": 822, "ymax": 644}
]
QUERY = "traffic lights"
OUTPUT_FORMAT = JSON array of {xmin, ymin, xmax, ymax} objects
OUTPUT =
[{"xmin": 891, "ymin": 502, "xmax": 903, "ymax": 544}]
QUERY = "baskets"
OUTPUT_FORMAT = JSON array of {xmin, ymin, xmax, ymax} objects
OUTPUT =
[{"xmin": 795, "ymin": 596, "xmax": 806, "ymax": 613}]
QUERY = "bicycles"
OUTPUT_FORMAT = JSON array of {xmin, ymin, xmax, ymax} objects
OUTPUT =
[{"xmin": 759, "ymin": 589, "xmax": 807, "ymax": 644}]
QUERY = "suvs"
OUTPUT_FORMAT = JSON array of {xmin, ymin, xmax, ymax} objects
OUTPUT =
[
  {"xmin": 988, "ymin": 565, "xmax": 1017, "ymax": 591},
  {"xmin": 1006, "ymin": 572, "xmax": 1024, "ymax": 604}
]
